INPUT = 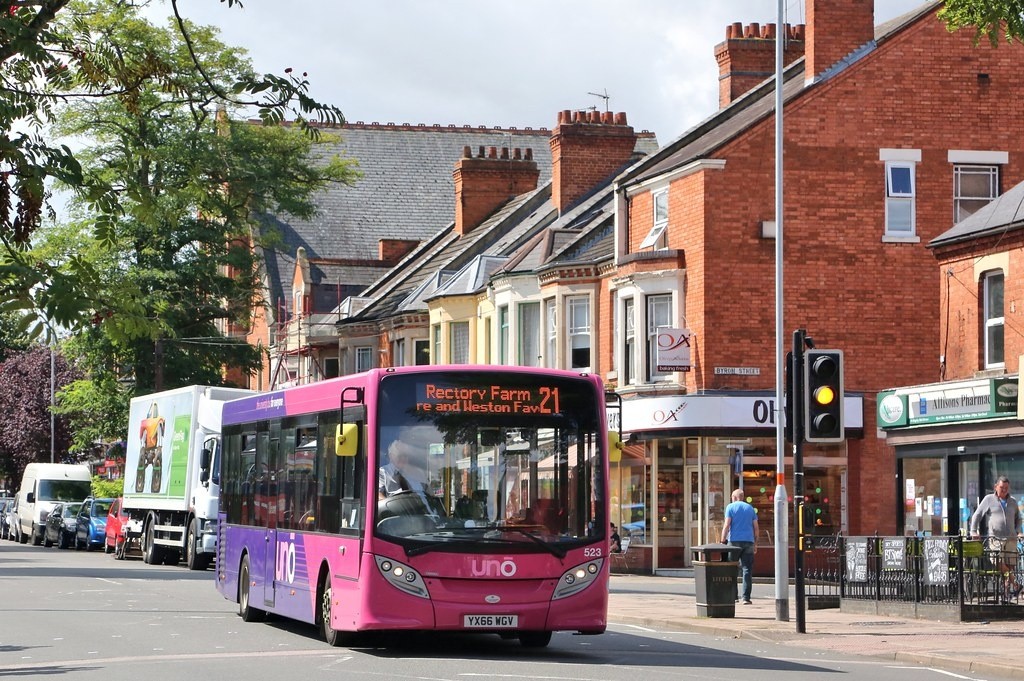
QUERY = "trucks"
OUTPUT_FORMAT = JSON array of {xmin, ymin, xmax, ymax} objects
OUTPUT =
[{"xmin": 121, "ymin": 383, "xmax": 269, "ymax": 571}]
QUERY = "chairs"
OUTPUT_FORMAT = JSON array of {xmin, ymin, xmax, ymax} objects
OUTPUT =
[
  {"xmin": 471, "ymin": 486, "xmax": 501, "ymax": 520},
  {"xmin": 879, "ymin": 538, "xmax": 1001, "ymax": 604},
  {"xmin": 94, "ymin": 505, "xmax": 104, "ymax": 516},
  {"xmin": 610, "ymin": 536, "xmax": 632, "ymax": 572}
]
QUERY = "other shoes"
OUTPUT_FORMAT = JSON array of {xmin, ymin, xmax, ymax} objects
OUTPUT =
[
  {"xmin": 742, "ymin": 598, "xmax": 752, "ymax": 605},
  {"xmin": 735, "ymin": 598, "xmax": 739, "ymax": 602}
]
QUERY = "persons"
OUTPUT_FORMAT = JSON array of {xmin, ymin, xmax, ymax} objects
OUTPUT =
[
  {"xmin": 378, "ymin": 440, "xmax": 434, "ymax": 500},
  {"xmin": 970, "ymin": 476, "xmax": 1024, "ymax": 605},
  {"xmin": 610, "ymin": 522, "xmax": 621, "ymax": 553},
  {"xmin": 721, "ymin": 489, "xmax": 759, "ymax": 604}
]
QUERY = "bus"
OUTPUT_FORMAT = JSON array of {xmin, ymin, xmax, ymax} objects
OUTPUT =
[{"xmin": 213, "ymin": 364, "xmax": 627, "ymax": 649}]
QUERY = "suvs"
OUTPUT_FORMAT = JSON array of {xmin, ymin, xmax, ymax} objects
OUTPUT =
[{"xmin": 75, "ymin": 495, "xmax": 119, "ymax": 551}]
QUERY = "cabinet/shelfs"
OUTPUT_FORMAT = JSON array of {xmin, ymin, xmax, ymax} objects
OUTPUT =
[{"xmin": 647, "ymin": 485, "xmax": 775, "ymax": 509}]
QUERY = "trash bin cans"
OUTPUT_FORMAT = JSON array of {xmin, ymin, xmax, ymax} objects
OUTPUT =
[{"xmin": 690, "ymin": 543, "xmax": 743, "ymax": 619}]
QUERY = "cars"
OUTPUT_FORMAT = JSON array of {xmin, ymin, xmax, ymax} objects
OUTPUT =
[
  {"xmin": 42, "ymin": 502, "xmax": 86, "ymax": 549},
  {"xmin": 0, "ymin": 488, "xmax": 21, "ymax": 543},
  {"xmin": 104, "ymin": 495, "xmax": 130, "ymax": 556}
]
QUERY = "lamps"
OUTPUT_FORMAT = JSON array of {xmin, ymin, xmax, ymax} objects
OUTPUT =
[{"xmin": 760, "ymin": 220, "xmax": 775, "ymax": 238}]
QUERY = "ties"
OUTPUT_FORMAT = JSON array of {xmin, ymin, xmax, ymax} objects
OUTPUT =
[{"xmin": 397, "ymin": 472, "xmax": 408, "ymax": 490}]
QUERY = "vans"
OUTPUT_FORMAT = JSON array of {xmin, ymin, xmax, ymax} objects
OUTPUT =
[{"xmin": 17, "ymin": 461, "xmax": 93, "ymax": 546}]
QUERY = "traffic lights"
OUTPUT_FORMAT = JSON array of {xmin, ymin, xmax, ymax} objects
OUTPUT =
[{"xmin": 803, "ymin": 345, "xmax": 850, "ymax": 444}]
